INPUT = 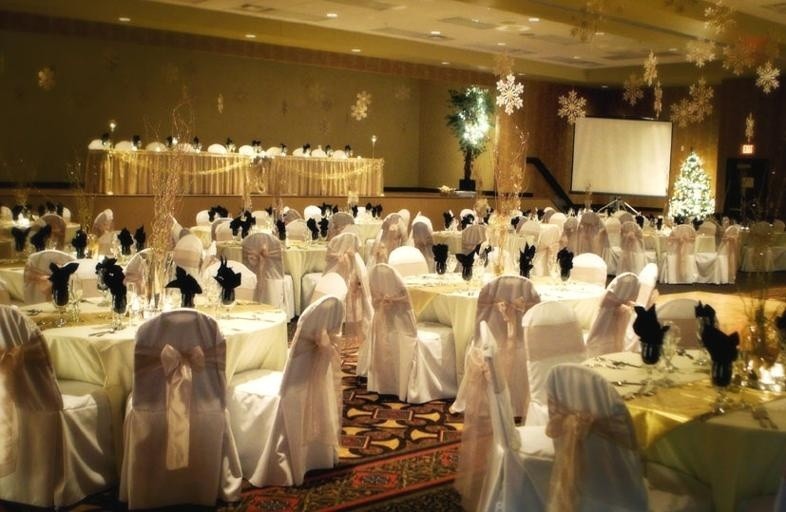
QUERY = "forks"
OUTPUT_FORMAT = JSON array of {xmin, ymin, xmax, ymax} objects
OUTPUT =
[{"xmin": 751, "ymin": 408, "xmax": 778, "ymax": 428}]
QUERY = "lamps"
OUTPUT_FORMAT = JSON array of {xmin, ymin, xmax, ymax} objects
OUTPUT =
[
  {"xmin": 370, "ymin": 131, "xmax": 378, "ymax": 159},
  {"xmin": 107, "ymin": 117, "xmax": 118, "ymax": 148}
]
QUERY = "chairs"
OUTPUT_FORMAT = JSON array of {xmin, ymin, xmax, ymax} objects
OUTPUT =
[{"xmin": 87, "ymin": 140, "xmax": 383, "ymax": 197}]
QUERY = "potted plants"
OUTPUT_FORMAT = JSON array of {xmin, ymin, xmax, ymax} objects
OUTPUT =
[{"xmin": 442, "ymin": 86, "xmax": 494, "ymax": 193}]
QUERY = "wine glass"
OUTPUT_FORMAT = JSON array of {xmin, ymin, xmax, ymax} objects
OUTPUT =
[
  {"xmin": 430, "ymin": 255, "xmax": 572, "ymax": 297},
  {"xmin": 50, "ymin": 271, "xmax": 237, "ymax": 331},
  {"xmin": 209, "ymin": 211, "xmax": 381, "ymax": 247},
  {"xmin": 10, "ymin": 204, "xmax": 138, "ymax": 266},
  {"xmin": 636, "ymin": 315, "xmax": 754, "ymax": 414}
]
